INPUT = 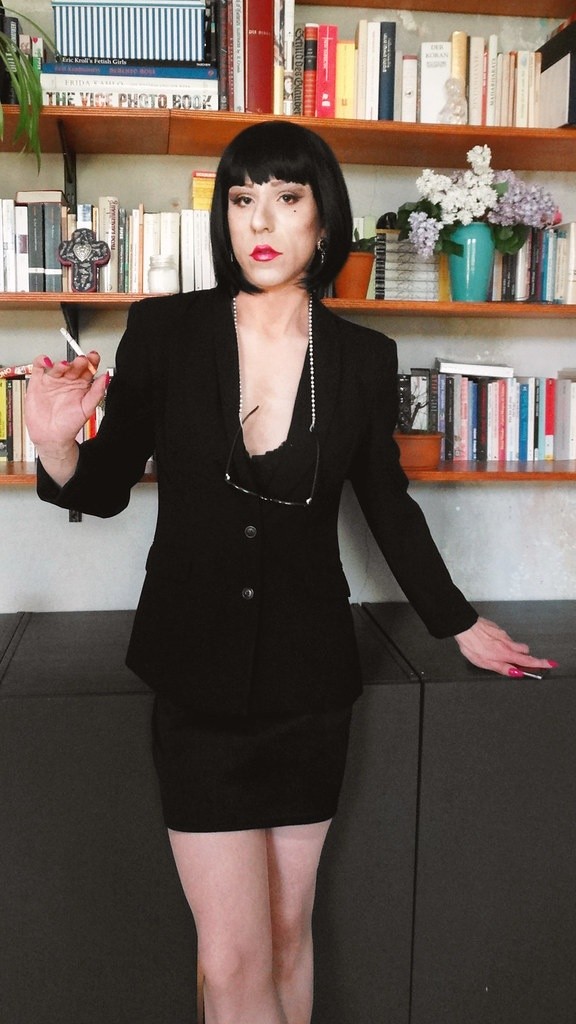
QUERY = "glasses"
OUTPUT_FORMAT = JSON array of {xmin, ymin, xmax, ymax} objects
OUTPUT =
[{"xmin": 225, "ymin": 405, "xmax": 320, "ymax": 510}]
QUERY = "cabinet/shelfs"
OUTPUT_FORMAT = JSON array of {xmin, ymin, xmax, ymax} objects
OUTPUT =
[
  {"xmin": 1, "ymin": 1, "xmax": 576, "ymax": 483},
  {"xmin": 2, "ymin": 596, "xmax": 575, "ymax": 1023}
]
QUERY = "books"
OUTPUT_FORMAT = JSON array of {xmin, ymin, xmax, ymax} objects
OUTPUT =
[{"xmin": 1, "ymin": 3, "xmax": 575, "ymax": 468}]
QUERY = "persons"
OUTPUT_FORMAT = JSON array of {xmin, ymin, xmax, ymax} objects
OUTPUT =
[{"xmin": 24, "ymin": 118, "xmax": 556, "ymax": 1024}]
